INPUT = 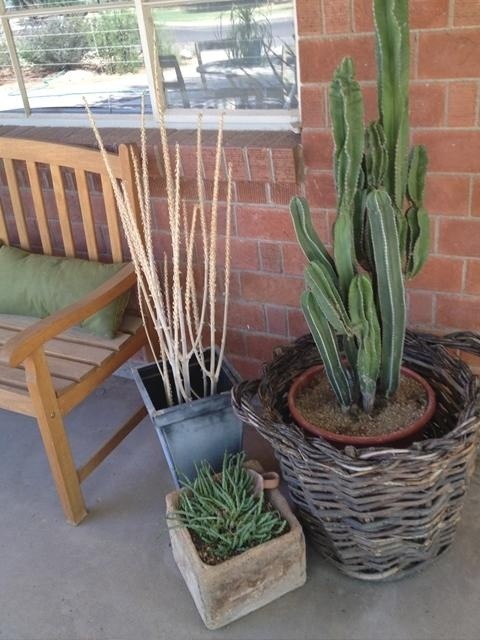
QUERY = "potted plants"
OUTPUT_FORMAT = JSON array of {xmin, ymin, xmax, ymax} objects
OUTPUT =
[
  {"xmin": 160, "ymin": 441, "xmax": 311, "ymax": 632},
  {"xmin": 78, "ymin": 23, "xmax": 258, "ymax": 491},
  {"xmin": 283, "ymin": 0, "xmax": 436, "ymax": 447}
]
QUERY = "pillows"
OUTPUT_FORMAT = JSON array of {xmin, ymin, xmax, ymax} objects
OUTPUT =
[{"xmin": 1, "ymin": 240, "xmax": 142, "ymax": 342}]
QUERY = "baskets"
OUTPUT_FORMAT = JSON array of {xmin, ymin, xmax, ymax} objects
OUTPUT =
[{"xmin": 230, "ymin": 324, "xmax": 479, "ymax": 585}]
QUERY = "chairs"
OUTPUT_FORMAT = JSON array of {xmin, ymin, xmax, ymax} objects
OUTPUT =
[{"xmin": 1, "ymin": 134, "xmax": 159, "ymax": 528}]
